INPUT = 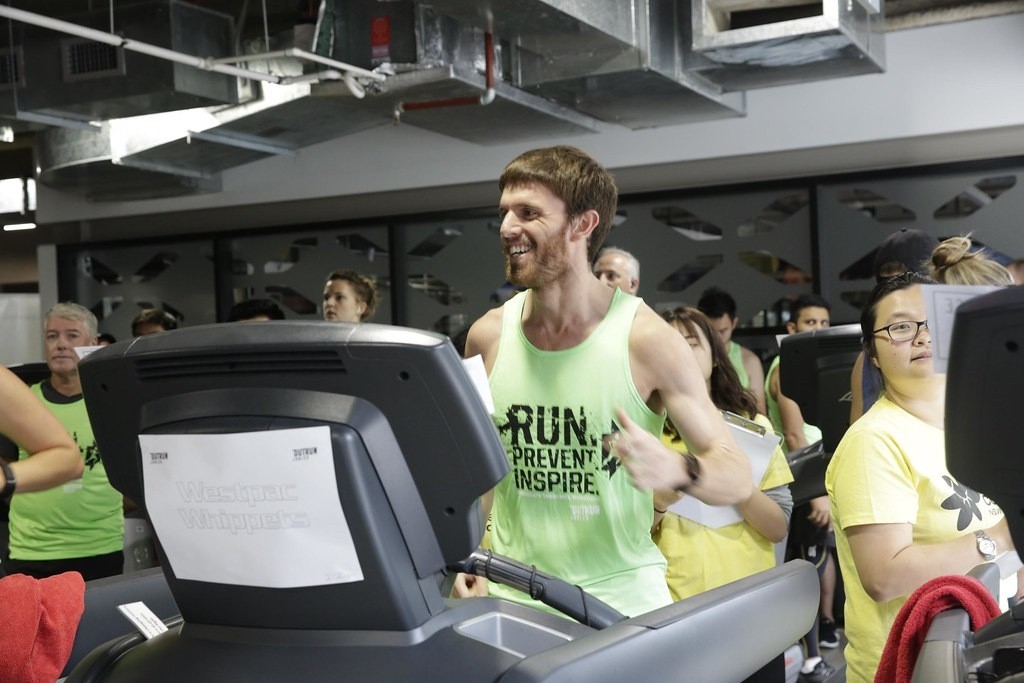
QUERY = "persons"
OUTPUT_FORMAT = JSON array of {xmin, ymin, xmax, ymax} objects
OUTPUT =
[
  {"xmin": 445, "ymin": 144, "xmax": 756, "ymax": 682},
  {"xmin": 322, "ymin": 270, "xmax": 376, "ymax": 323},
  {"xmin": 3, "ymin": 299, "xmax": 131, "ymax": 590},
  {"xmin": 906, "ymin": 235, "xmax": 1024, "ymax": 601},
  {"xmin": 762, "ymin": 292, "xmax": 846, "ymax": 649},
  {"xmin": 0, "ymin": 357, "xmax": 88, "ymax": 583},
  {"xmin": 592, "ymin": 245, "xmax": 641, "ymax": 305},
  {"xmin": 649, "ymin": 303, "xmax": 798, "ymax": 683},
  {"xmin": 821, "ymin": 274, "xmax": 1024, "ymax": 683},
  {"xmin": 694, "ymin": 285, "xmax": 764, "ymax": 435},
  {"xmin": 223, "ymin": 298, "xmax": 289, "ymax": 330},
  {"xmin": 93, "ymin": 331, "xmax": 119, "ymax": 347},
  {"xmin": 129, "ymin": 309, "xmax": 180, "ymax": 337}
]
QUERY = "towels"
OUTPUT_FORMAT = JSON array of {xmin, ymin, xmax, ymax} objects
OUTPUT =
[{"xmin": 874, "ymin": 575, "xmax": 1003, "ymax": 683}]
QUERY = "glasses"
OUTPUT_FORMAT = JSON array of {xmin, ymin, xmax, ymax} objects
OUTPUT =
[{"xmin": 870, "ymin": 320, "xmax": 929, "ymax": 342}]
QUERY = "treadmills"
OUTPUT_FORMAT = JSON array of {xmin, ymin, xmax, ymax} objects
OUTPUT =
[
  {"xmin": 63, "ymin": 320, "xmax": 821, "ymax": 683},
  {"xmin": 779, "ymin": 322, "xmax": 864, "ymax": 508},
  {"xmin": 908, "ymin": 282, "xmax": 1024, "ymax": 683}
]
